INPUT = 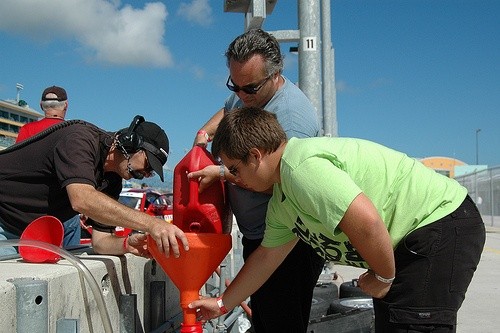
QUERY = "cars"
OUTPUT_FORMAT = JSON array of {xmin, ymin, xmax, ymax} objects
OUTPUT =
[{"xmin": 107, "ymin": 189, "xmax": 174, "ymax": 234}]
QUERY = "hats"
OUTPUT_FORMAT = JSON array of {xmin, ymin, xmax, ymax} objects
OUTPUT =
[
  {"xmin": 132, "ymin": 120, "xmax": 170, "ymax": 183},
  {"xmin": 42, "ymin": 85, "xmax": 68, "ymax": 101}
]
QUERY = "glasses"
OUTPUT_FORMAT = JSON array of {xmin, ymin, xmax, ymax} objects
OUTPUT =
[
  {"xmin": 229, "ymin": 152, "xmax": 250, "ymax": 177},
  {"xmin": 226, "ymin": 73, "xmax": 272, "ymax": 94}
]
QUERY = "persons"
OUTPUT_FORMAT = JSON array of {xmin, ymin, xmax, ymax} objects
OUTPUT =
[
  {"xmin": 187, "ymin": 28, "xmax": 326, "ymax": 333},
  {"xmin": 15, "ymin": 86, "xmax": 81, "ymax": 249},
  {"xmin": 0, "ymin": 115, "xmax": 191, "ymax": 260},
  {"xmin": 188, "ymin": 106, "xmax": 486, "ymax": 333}
]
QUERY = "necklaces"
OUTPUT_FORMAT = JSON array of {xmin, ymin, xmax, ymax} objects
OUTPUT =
[{"xmin": 46, "ymin": 115, "xmax": 64, "ymax": 118}]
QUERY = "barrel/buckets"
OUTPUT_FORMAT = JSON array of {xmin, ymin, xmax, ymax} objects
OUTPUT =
[{"xmin": 173, "ymin": 146, "xmax": 233, "ymax": 234}]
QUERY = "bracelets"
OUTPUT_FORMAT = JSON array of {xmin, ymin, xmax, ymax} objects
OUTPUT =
[
  {"xmin": 216, "ymin": 297, "xmax": 228, "ymax": 314},
  {"xmin": 375, "ymin": 273, "xmax": 395, "ymax": 284},
  {"xmin": 123, "ymin": 236, "xmax": 127, "ymax": 252},
  {"xmin": 220, "ymin": 165, "xmax": 224, "ymax": 180},
  {"xmin": 197, "ymin": 130, "xmax": 210, "ymax": 142}
]
